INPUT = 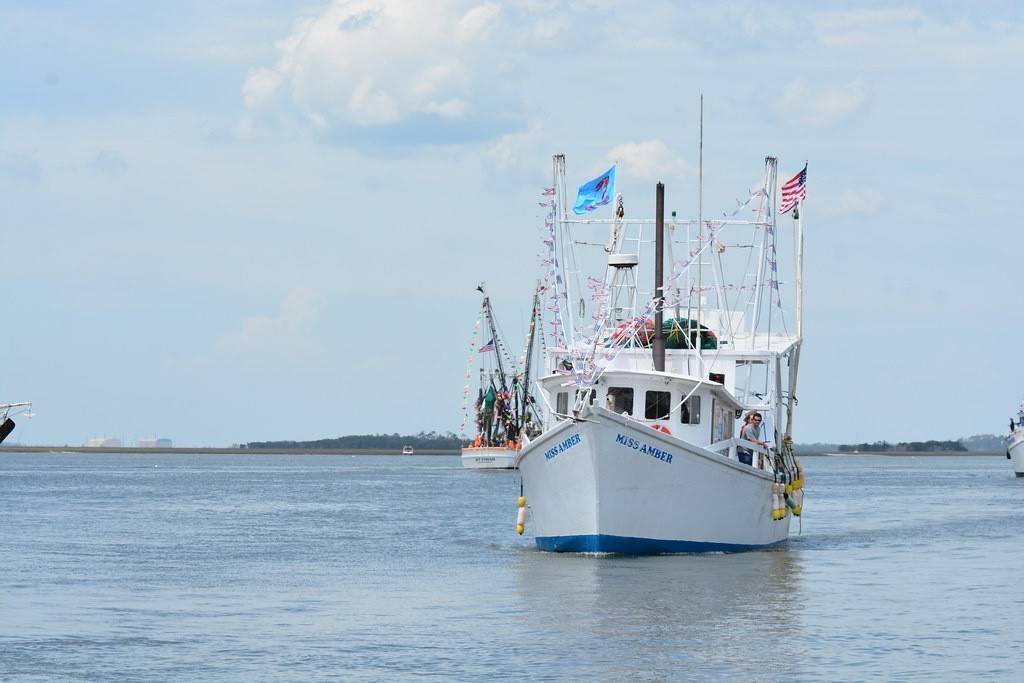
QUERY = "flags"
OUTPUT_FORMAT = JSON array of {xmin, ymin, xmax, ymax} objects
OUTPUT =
[
  {"xmin": 574, "ymin": 165, "xmax": 615, "ymax": 214},
  {"xmin": 540, "ymin": 187, "xmax": 781, "ymax": 386},
  {"xmin": 479, "ymin": 339, "xmax": 494, "ymax": 353},
  {"xmin": 778, "ymin": 167, "xmax": 806, "ymax": 214}
]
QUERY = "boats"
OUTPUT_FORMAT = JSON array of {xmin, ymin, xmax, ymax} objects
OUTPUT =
[
  {"xmin": 512, "ymin": 90, "xmax": 810, "ymax": 555},
  {"xmin": 459, "ymin": 282, "xmax": 546, "ymax": 471},
  {"xmin": 1002, "ymin": 402, "xmax": 1024, "ymax": 480},
  {"xmin": 401, "ymin": 445, "xmax": 414, "ymax": 456},
  {"xmin": 0, "ymin": 400, "xmax": 39, "ymax": 443}
]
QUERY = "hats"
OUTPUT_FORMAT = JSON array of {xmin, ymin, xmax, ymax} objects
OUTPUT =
[{"xmin": 743, "ymin": 409, "xmax": 757, "ymax": 421}]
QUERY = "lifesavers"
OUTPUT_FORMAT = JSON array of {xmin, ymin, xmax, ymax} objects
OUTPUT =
[{"xmin": 651, "ymin": 424, "xmax": 672, "ymax": 434}]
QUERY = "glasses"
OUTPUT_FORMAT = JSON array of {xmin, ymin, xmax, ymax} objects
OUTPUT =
[{"xmin": 754, "ymin": 419, "xmax": 761, "ymax": 422}]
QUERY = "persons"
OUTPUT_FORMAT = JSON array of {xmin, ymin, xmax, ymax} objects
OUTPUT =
[
  {"xmin": 1008, "ymin": 418, "xmax": 1014, "ymax": 431},
  {"xmin": 738, "ymin": 413, "xmax": 768, "ymax": 466},
  {"xmin": 740, "ymin": 414, "xmax": 754, "ymax": 438}
]
